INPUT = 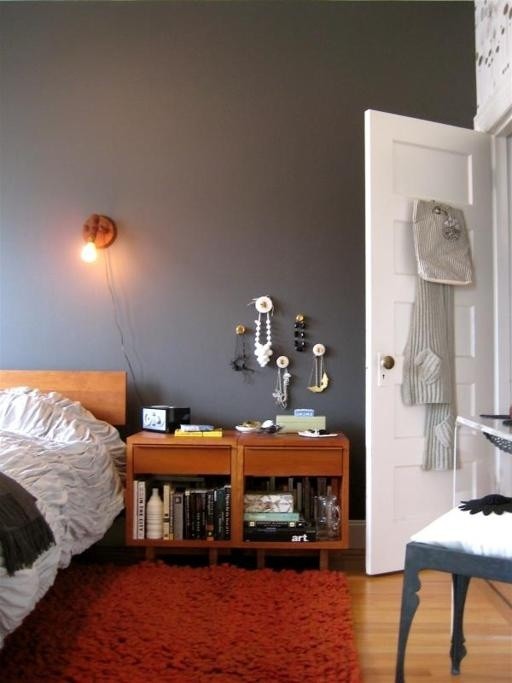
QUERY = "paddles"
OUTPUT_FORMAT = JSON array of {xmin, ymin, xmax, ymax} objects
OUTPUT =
[{"xmin": 0, "ymin": 563, "xmax": 362, "ymax": 683}]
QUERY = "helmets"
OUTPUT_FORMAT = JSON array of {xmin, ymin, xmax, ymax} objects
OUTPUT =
[
  {"xmin": 299, "ymin": 431, "xmax": 337, "ymax": 438},
  {"xmin": 234, "ymin": 424, "xmax": 258, "ymax": 433}
]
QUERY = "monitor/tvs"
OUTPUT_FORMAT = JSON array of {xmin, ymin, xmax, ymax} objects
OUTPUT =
[
  {"xmin": 174, "ymin": 427, "xmax": 224, "ymax": 438},
  {"xmin": 133, "ymin": 478, "xmax": 152, "ymax": 541},
  {"xmin": 244, "ymin": 476, "xmax": 332, "ymax": 540},
  {"xmin": 162, "ymin": 480, "xmax": 232, "ymax": 541}
]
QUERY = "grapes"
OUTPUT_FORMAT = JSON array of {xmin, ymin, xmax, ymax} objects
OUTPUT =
[
  {"xmin": 128, "ymin": 427, "xmax": 235, "ymax": 565},
  {"xmin": 237, "ymin": 430, "xmax": 350, "ymax": 568}
]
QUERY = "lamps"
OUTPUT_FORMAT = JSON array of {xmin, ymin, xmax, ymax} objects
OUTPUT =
[{"xmin": 396, "ymin": 416, "xmax": 512, "ymax": 683}]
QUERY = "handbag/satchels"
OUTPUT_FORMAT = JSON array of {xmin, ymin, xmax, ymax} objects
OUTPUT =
[{"xmin": 293, "ymin": 313, "xmax": 306, "ymax": 352}]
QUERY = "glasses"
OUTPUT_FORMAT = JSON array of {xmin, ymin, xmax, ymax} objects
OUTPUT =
[{"xmin": 81, "ymin": 214, "xmax": 100, "ymax": 264}]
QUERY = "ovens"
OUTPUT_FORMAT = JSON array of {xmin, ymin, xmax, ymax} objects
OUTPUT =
[{"xmin": 0, "ymin": 369, "xmax": 127, "ymax": 641}]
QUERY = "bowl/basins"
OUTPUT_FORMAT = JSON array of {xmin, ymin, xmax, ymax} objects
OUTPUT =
[
  {"xmin": 306, "ymin": 353, "xmax": 329, "ymax": 393},
  {"xmin": 248, "ymin": 294, "xmax": 274, "ymax": 367},
  {"xmin": 230, "ymin": 335, "xmax": 248, "ymax": 371},
  {"xmin": 273, "ymin": 367, "xmax": 291, "ymax": 408}
]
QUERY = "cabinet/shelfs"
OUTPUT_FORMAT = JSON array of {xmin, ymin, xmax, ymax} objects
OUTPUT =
[{"xmin": 146, "ymin": 488, "xmax": 163, "ymax": 539}]
